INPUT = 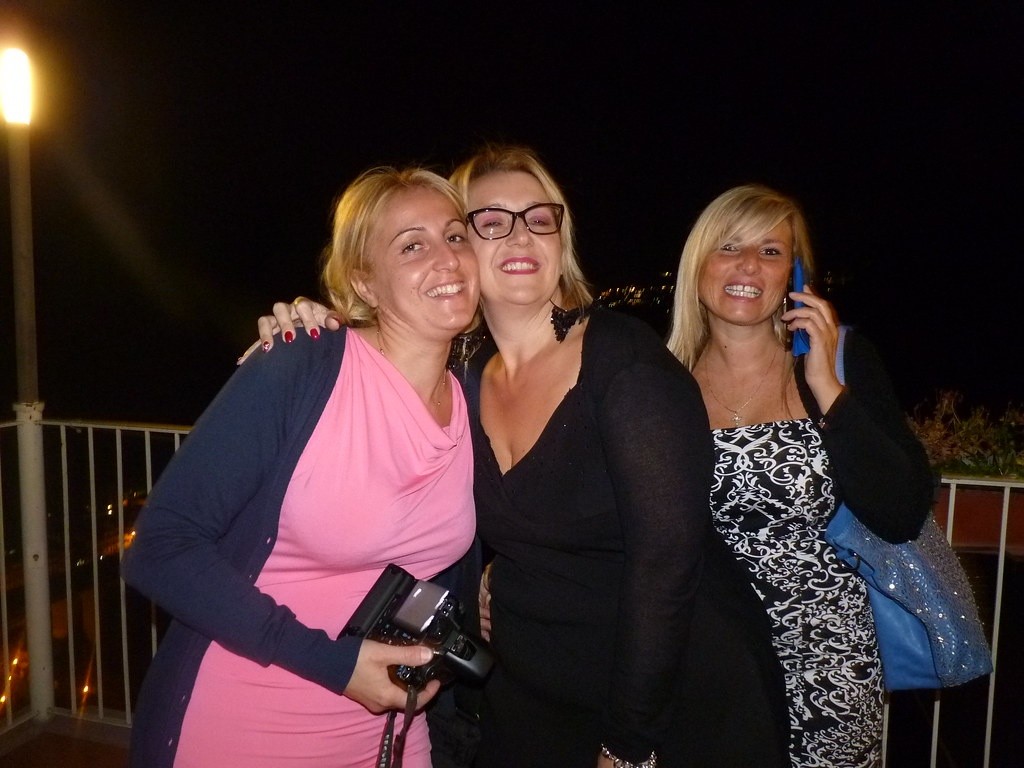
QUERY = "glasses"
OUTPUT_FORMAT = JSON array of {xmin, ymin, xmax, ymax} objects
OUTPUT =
[{"xmin": 464, "ymin": 203, "xmax": 564, "ymax": 240}]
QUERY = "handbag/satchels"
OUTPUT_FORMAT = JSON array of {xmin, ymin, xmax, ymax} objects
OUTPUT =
[{"xmin": 824, "ymin": 325, "xmax": 994, "ymax": 695}]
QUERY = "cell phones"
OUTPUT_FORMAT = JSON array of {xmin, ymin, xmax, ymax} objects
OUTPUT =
[{"xmin": 787, "ymin": 275, "xmax": 795, "ymax": 350}]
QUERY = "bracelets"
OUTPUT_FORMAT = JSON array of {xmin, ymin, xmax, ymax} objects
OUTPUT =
[{"xmin": 598, "ymin": 742, "xmax": 657, "ymax": 768}]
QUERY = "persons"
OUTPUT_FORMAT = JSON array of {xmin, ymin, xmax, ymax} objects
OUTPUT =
[
  {"xmin": 479, "ymin": 183, "xmax": 943, "ymax": 768},
  {"xmin": 235, "ymin": 145, "xmax": 797, "ymax": 768},
  {"xmin": 120, "ymin": 166, "xmax": 481, "ymax": 768}
]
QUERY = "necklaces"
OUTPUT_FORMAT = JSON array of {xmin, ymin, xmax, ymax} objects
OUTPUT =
[
  {"xmin": 375, "ymin": 327, "xmax": 447, "ymax": 420},
  {"xmin": 705, "ymin": 349, "xmax": 777, "ymax": 428}
]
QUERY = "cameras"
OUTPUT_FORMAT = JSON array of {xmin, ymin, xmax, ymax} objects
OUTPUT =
[{"xmin": 338, "ymin": 564, "xmax": 499, "ymax": 705}]
